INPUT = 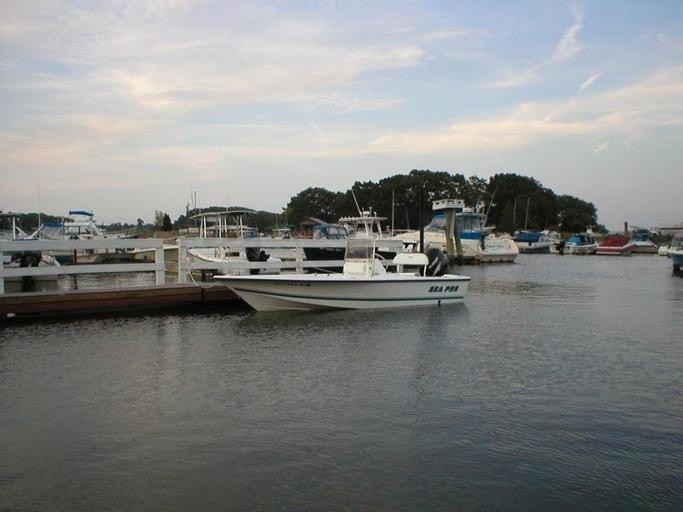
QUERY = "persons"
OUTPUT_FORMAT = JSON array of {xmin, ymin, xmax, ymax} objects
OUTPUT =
[{"xmin": 19, "ymin": 250, "xmax": 41, "ymax": 292}]
